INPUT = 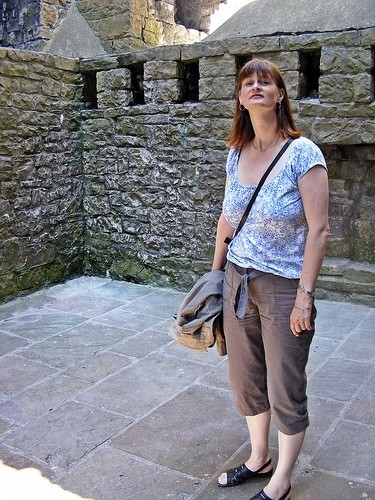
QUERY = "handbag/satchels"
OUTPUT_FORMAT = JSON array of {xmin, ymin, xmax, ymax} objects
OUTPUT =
[{"xmin": 208, "ymin": 305, "xmax": 231, "ymax": 358}]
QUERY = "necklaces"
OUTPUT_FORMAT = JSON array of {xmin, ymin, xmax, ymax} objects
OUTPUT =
[{"xmin": 253, "ymin": 132, "xmax": 282, "ymax": 152}]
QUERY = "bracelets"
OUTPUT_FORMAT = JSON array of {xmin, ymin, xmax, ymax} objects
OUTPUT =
[{"xmin": 297, "ymin": 285, "xmax": 313, "ymax": 296}]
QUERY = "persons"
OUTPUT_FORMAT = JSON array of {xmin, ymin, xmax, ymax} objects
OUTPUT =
[{"xmin": 211, "ymin": 59, "xmax": 331, "ymax": 500}]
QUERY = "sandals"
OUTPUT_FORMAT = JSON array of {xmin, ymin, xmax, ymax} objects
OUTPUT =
[
  {"xmin": 250, "ymin": 483, "xmax": 292, "ymax": 499},
  {"xmin": 216, "ymin": 455, "xmax": 273, "ymax": 487}
]
